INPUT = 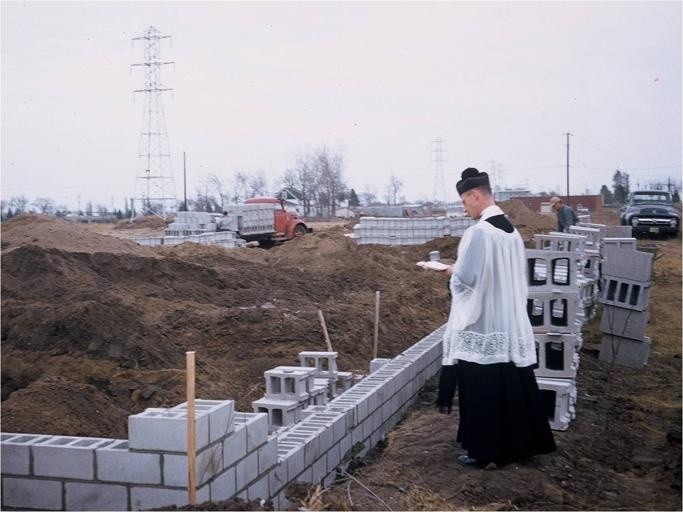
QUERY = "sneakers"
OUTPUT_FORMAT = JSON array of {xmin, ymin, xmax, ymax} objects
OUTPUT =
[{"xmin": 456, "ymin": 454, "xmax": 479, "ymax": 467}]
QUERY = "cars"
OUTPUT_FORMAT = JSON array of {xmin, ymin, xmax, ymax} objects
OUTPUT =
[{"xmin": 621, "ymin": 191, "xmax": 681, "ymax": 239}]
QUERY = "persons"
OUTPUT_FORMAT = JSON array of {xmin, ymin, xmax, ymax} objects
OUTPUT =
[
  {"xmin": 219, "ymin": 211, "xmax": 232, "ymax": 231},
  {"xmin": 547, "ymin": 197, "xmax": 579, "ymax": 232},
  {"xmin": 429, "ymin": 167, "xmax": 556, "ymax": 472}
]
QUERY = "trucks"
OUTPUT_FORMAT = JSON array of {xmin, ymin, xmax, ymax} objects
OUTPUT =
[{"xmin": 240, "ymin": 198, "xmax": 307, "ymax": 242}]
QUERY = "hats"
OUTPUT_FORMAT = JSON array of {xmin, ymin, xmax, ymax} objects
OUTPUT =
[
  {"xmin": 549, "ymin": 197, "xmax": 563, "ymax": 208},
  {"xmin": 455, "ymin": 167, "xmax": 490, "ymax": 196}
]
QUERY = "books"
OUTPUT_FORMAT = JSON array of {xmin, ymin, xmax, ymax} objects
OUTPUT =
[{"xmin": 415, "ymin": 258, "xmax": 449, "ymax": 272}]
